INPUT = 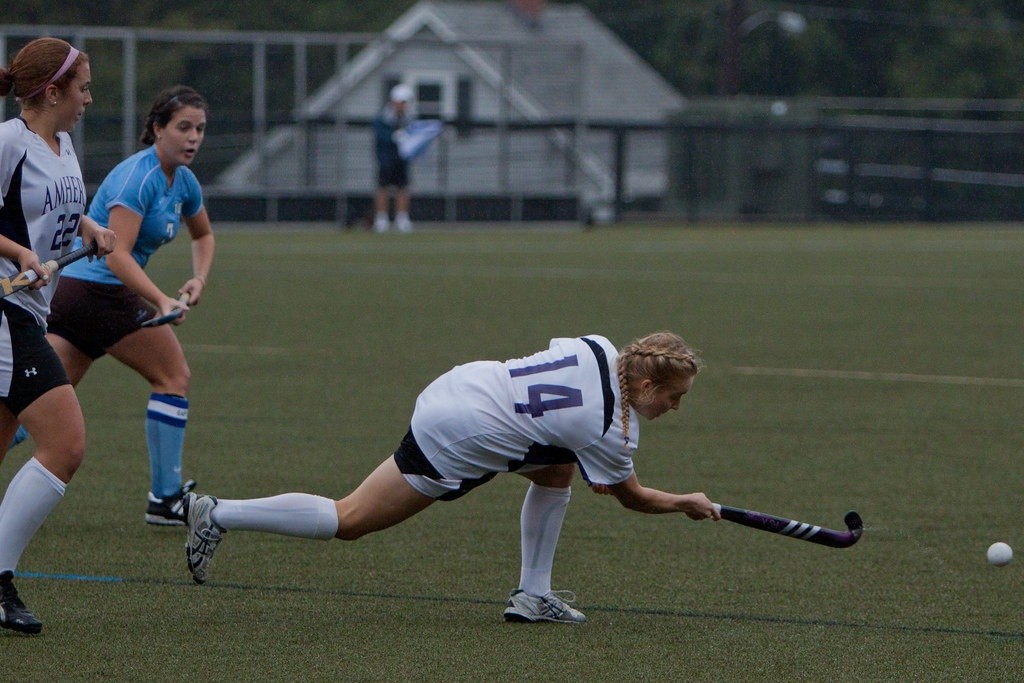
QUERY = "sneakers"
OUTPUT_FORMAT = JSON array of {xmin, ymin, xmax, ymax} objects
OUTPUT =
[
  {"xmin": 504, "ymin": 588, "xmax": 587, "ymax": 624},
  {"xmin": 0, "ymin": 570, "xmax": 43, "ymax": 634},
  {"xmin": 182, "ymin": 492, "xmax": 226, "ymax": 584},
  {"xmin": 146, "ymin": 479, "xmax": 197, "ymax": 526}
]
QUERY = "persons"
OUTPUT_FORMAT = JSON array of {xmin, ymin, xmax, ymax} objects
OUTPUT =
[
  {"xmin": 0, "ymin": 38, "xmax": 94, "ymax": 632},
  {"xmin": 184, "ymin": 332, "xmax": 721, "ymax": 624},
  {"xmin": 372, "ymin": 84, "xmax": 414, "ymax": 232},
  {"xmin": 7, "ymin": 86, "xmax": 216, "ymax": 525}
]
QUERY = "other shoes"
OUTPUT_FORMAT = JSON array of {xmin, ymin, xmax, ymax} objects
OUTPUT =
[{"xmin": 374, "ymin": 216, "xmax": 413, "ymax": 233}]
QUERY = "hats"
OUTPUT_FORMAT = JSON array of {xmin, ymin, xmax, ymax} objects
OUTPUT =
[{"xmin": 390, "ymin": 84, "xmax": 412, "ymax": 103}]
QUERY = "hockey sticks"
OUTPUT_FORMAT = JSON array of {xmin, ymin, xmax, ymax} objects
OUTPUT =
[
  {"xmin": 140, "ymin": 292, "xmax": 190, "ymax": 328},
  {"xmin": 1, "ymin": 240, "xmax": 98, "ymax": 299},
  {"xmin": 710, "ymin": 502, "xmax": 864, "ymax": 548}
]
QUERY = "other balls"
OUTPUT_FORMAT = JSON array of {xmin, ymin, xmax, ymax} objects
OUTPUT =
[{"xmin": 988, "ymin": 542, "xmax": 1013, "ymax": 567}]
